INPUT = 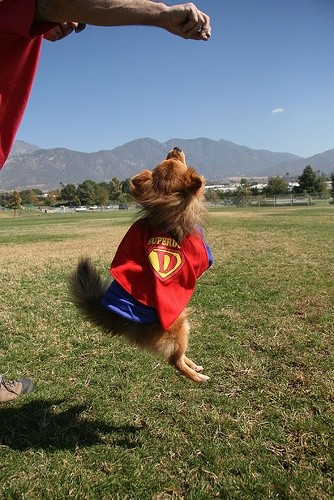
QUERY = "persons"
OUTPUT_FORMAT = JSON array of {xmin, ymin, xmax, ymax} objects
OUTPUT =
[{"xmin": 0, "ymin": 0, "xmax": 211, "ymax": 404}]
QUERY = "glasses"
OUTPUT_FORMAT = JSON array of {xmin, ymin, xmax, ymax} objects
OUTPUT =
[{"xmin": 75, "ymin": 22, "xmax": 86, "ymax": 33}]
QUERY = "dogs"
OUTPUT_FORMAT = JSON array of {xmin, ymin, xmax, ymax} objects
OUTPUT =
[{"xmin": 64, "ymin": 147, "xmax": 213, "ymax": 383}]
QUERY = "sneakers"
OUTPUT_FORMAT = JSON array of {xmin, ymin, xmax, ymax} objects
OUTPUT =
[{"xmin": 0, "ymin": 378, "xmax": 34, "ymax": 403}]
